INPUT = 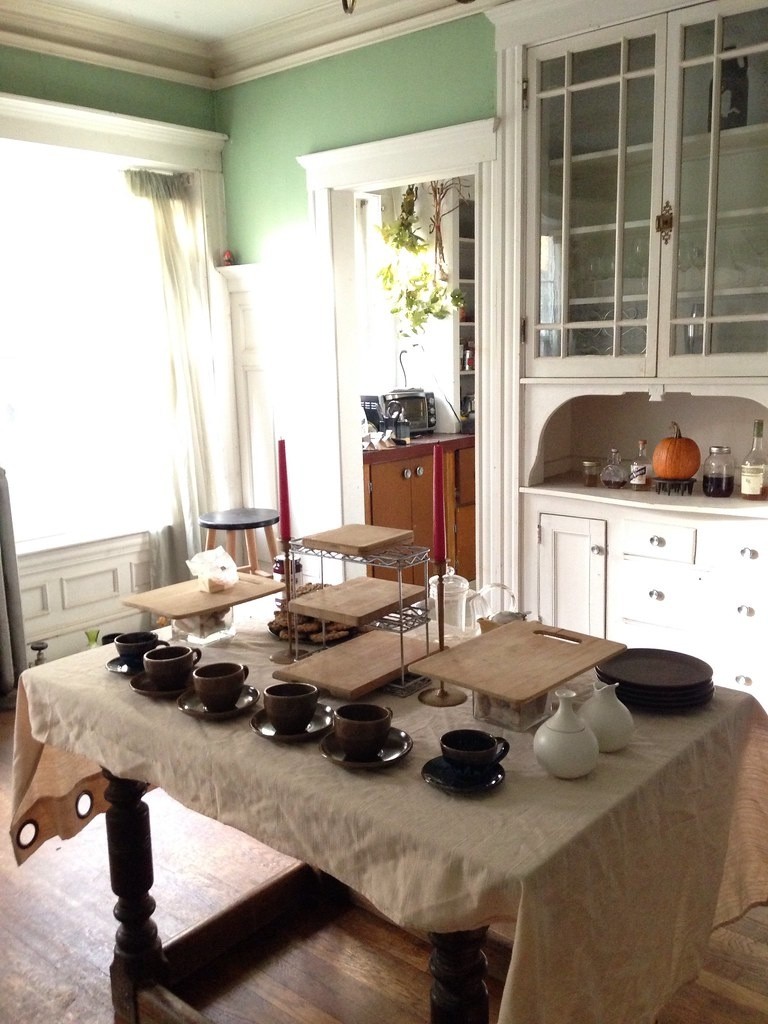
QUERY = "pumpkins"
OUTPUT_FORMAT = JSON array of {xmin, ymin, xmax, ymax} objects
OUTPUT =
[{"xmin": 653, "ymin": 421, "xmax": 701, "ymax": 480}]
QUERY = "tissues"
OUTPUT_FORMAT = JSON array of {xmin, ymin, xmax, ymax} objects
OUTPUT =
[{"xmin": 183, "ymin": 544, "xmax": 241, "ymax": 593}]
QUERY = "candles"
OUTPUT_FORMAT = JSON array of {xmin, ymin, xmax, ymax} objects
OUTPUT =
[
  {"xmin": 432, "ymin": 440, "xmax": 447, "ymax": 562},
  {"xmin": 277, "ymin": 440, "xmax": 292, "ymax": 538}
]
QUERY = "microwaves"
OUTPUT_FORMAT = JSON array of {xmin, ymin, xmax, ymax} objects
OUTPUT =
[{"xmin": 361, "ymin": 388, "xmax": 437, "ymax": 439}]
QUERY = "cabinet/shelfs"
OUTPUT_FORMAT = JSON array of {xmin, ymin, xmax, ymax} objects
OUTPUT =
[
  {"xmin": 485, "ymin": 0, "xmax": 768, "ymax": 719},
  {"xmin": 364, "ymin": 183, "xmax": 475, "ymax": 587}
]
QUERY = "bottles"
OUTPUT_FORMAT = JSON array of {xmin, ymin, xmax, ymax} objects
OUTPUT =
[
  {"xmin": 565, "ymin": 304, "xmax": 768, "ymax": 500},
  {"xmin": 578, "ymin": 682, "xmax": 633, "ymax": 754},
  {"xmin": 272, "ymin": 555, "xmax": 303, "ymax": 607},
  {"xmin": 533, "ymin": 689, "xmax": 598, "ymax": 778},
  {"xmin": 708, "ymin": 46, "xmax": 749, "ymax": 132},
  {"xmin": 456, "ymin": 297, "xmax": 476, "ymax": 434},
  {"xmin": 378, "ymin": 407, "xmax": 410, "ymax": 446}
]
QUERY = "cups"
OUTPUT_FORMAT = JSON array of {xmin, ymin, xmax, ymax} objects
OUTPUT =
[
  {"xmin": 334, "ymin": 703, "xmax": 393, "ymax": 757},
  {"xmin": 430, "ymin": 566, "xmax": 489, "ymax": 643},
  {"xmin": 114, "ymin": 631, "xmax": 170, "ymax": 669},
  {"xmin": 144, "ymin": 646, "xmax": 201, "ymax": 689},
  {"xmin": 439, "ymin": 729, "xmax": 510, "ymax": 784},
  {"xmin": 192, "ymin": 662, "xmax": 249, "ymax": 712},
  {"xmin": 263, "ymin": 682, "xmax": 320, "ymax": 735}
]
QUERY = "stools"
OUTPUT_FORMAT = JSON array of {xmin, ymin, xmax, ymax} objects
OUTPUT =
[{"xmin": 200, "ymin": 507, "xmax": 279, "ymax": 573}]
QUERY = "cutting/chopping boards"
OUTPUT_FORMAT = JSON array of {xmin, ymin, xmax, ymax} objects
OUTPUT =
[{"xmin": 120, "ymin": 524, "xmax": 627, "ymax": 700}]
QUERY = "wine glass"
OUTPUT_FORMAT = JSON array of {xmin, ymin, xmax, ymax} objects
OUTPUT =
[{"xmin": 573, "ymin": 220, "xmax": 768, "ymax": 296}]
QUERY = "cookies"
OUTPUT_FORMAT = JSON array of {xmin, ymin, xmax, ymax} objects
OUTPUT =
[{"xmin": 273, "ymin": 583, "xmax": 359, "ymax": 642}]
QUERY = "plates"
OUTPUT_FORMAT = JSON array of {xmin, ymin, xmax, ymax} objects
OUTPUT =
[
  {"xmin": 595, "ymin": 648, "xmax": 715, "ymax": 713},
  {"xmin": 106, "ymin": 656, "xmax": 506, "ymax": 795}
]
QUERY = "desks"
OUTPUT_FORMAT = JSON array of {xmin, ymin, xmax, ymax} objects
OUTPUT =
[{"xmin": 11, "ymin": 596, "xmax": 768, "ymax": 1024}]
